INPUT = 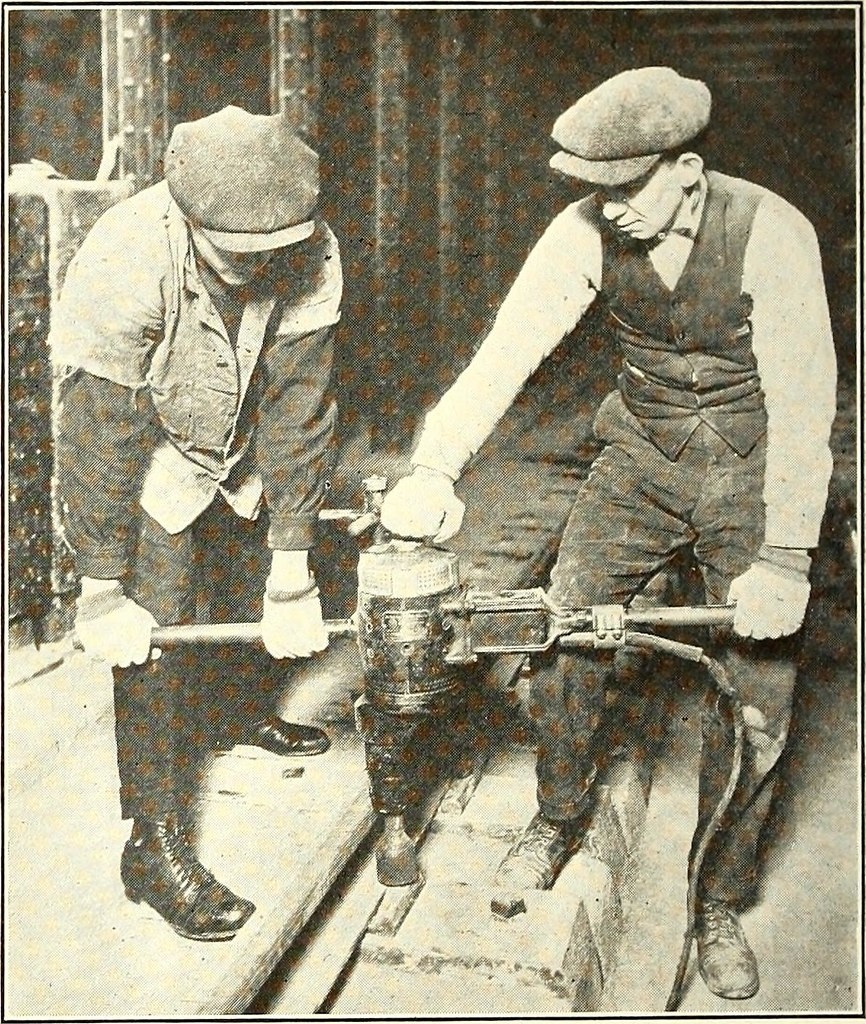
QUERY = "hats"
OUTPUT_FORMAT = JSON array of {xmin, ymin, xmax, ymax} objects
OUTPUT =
[
  {"xmin": 162, "ymin": 103, "xmax": 323, "ymax": 254},
  {"xmin": 548, "ymin": 66, "xmax": 713, "ymax": 185}
]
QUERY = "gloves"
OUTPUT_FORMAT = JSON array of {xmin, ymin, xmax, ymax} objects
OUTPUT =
[
  {"xmin": 379, "ymin": 471, "xmax": 467, "ymax": 543},
  {"xmin": 724, "ymin": 543, "xmax": 812, "ymax": 641},
  {"xmin": 260, "ymin": 570, "xmax": 329, "ymax": 660},
  {"xmin": 73, "ymin": 583, "xmax": 162, "ymax": 668}
]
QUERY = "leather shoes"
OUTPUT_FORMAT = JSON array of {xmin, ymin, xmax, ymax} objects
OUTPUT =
[
  {"xmin": 694, "ymin": 897, "xmax": 760, "ymax": 1000},
  {"xmin": 237, "ymin": 716, "xmax": 328, "ymax": 757},
  {"xmin": 489, "ymin": 808, "xmax": 586, "ymax": 922},
  {"xmin": 120, "ymin": 828, "xmax": 256, "ymax": 941}
]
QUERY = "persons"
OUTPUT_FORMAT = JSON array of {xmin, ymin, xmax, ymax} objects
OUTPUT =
[
  {"xmin": 52, "ymin": 106, "xmax": 347, "ymax": 941},
  {"xmin": 381, "ymin": 68, "xmax": 839, "ymax": 999}
]
